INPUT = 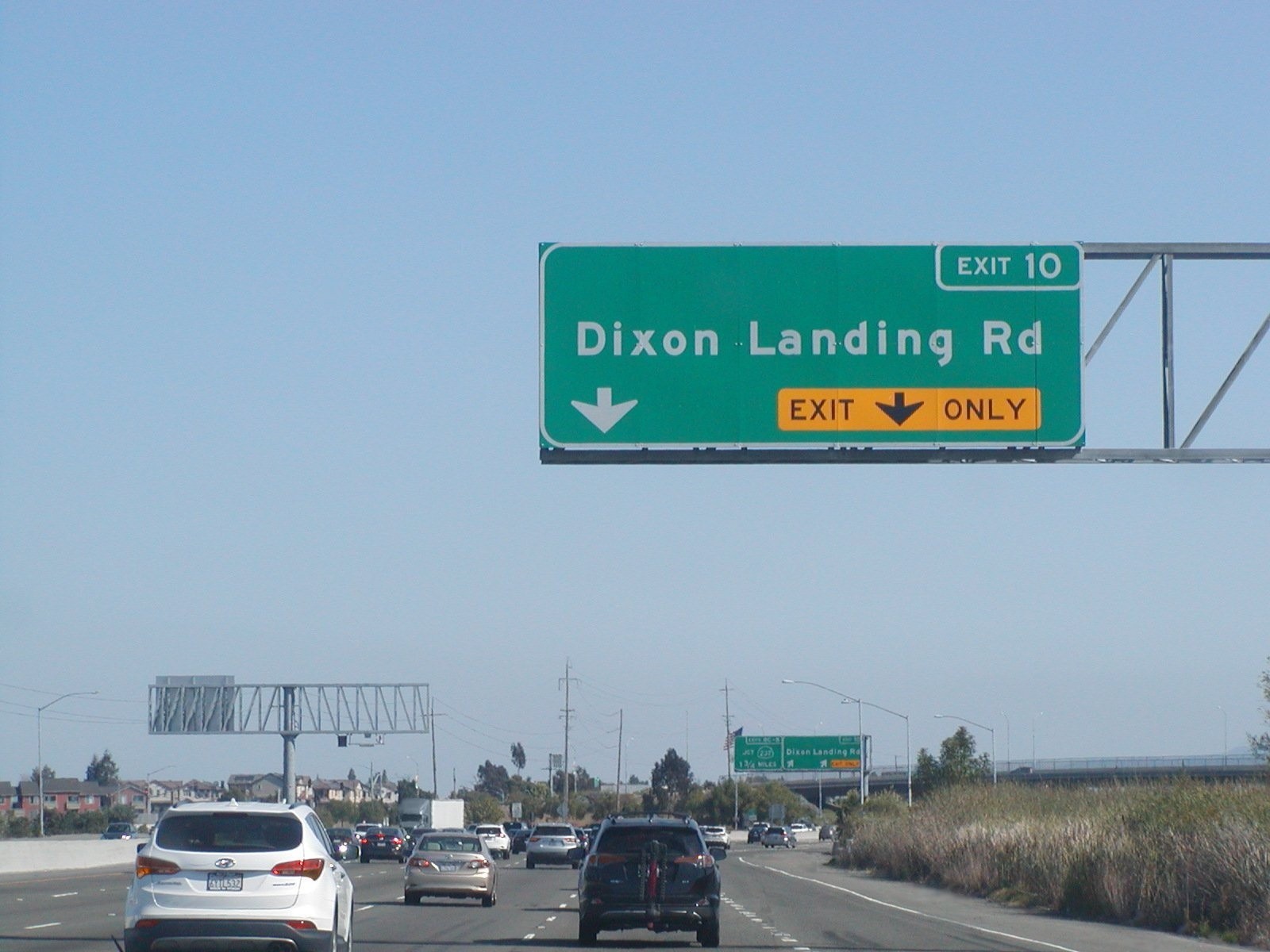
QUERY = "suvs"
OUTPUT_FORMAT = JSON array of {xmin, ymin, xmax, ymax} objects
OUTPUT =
[
  {"xmin": 572, "ymin": 816, "xmax": 724, "ymax": 948},
  {"xmin": 699, "ymin": 824, "xmax": 731, "ymax": 849},
  {"xmin": 121, "ymin": 799, "xmax": 357, "ymax": 952}
]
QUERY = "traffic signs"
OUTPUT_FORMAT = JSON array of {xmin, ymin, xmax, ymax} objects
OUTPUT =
[
  {"xmin": 536, "ymin": 239, "xmax": 1094, "ymax": 462},
  {"xmin": 734, "ymin": 733, "xmax": 870, "ymax": 772}
]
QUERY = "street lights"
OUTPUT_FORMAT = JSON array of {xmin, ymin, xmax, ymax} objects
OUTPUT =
[
  {"xmin": 781, "ymin": 678, "xmax": 864, "ymax": 811},
  {"xmin": 841, "ymin": 697, "xmax": 913, "ymax": 809},
  {"xmin": 35, "ymin": 689, "xmax": 100, "ymax": 838},
  {"xmin": 934, "ymin": 714, "xmax": 998, "ymax": 788}
]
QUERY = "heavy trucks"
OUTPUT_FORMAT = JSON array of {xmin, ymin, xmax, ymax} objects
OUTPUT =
[{"xmin": 396, "ymin": 797, "xmax": 468, "ymax": 839}]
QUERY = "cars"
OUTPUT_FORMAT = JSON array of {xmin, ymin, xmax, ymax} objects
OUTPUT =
[
  {"xmin": 324, "ymin": 826, "xmax": 357, "ymax": 860},
  {"xmin": 504, "ymin": 820, "xmax": 525, "ymax": 840},
  {"xmin": 748, "ymin": 821, "xmax": 838, "ymax": 852},
  {"xmin": 363, "ymin": 828, "xmax": 410, "ymax": 863},
  {"xmin": 354, "ymin": 822, "xmax": 381, "ymax": 846},
  {"xmin": 401, "ymin": 831, "xmax": 499, "ymax": 906},
  {"xmin": 527, "ymin": 824, "xmax": 583, "ymax": 872},
  {"xmin": 409, "ymin": 827, "xmax": 437, "ymax": 849},
  {"xmin": 468, "ymin": 822, "xmax": 511, "ymax": 861},
  {"xmin": 100, "ymin": 823, "xmax": 137, "ymax": 841},
  {"xmin": 574, "ymin": 828, "xmax": 591, "ymax": 856},
  {"xmin": 511, "ymin": 829, "xmax": 533, "ymax": 854}
]
